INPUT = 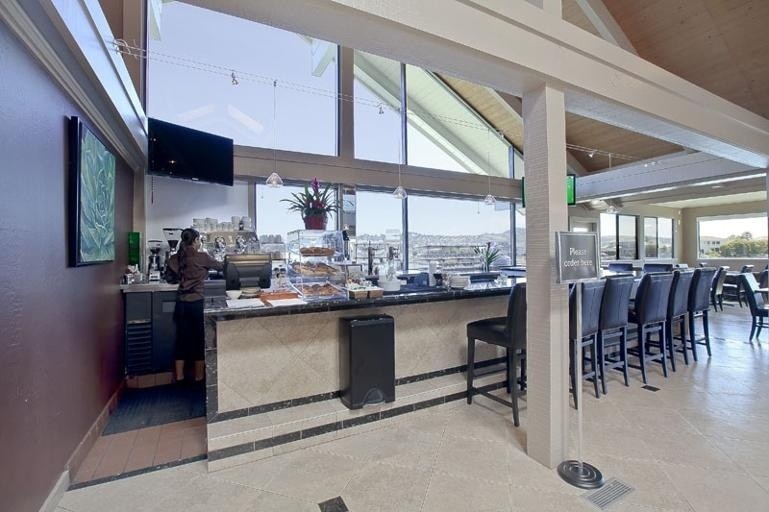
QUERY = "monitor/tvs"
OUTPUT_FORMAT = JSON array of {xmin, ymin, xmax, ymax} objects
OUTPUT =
[
  {"xmin": 522, "ymin": 174, "xmax": 576, "ymax": 208},
  {"xmin": 224, "ymin": 254, "xmax": 272, "ymax": 290},
  {"xmin": 147, "ymin": 117, "xmax": 234, "ymax": 186}
]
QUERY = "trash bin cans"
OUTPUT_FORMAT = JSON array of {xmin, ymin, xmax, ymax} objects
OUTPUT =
[{"xmin": 338, "ymin": 313, "xmax": 395, "ymax": 410}]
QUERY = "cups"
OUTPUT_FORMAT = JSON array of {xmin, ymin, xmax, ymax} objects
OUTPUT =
[
  {"xmin": 195, "ymin": 223, "xmax": 234, "ymax": 232},
  {"xmin": 501, "ymin": 275, "xmax": 508, "ymax": 285},
  {"xmin": 260, "ymin": 234, "xmax": 282, "ymax": 243},
  {"xmin": 230, "ymin": 215, "xmax": 254, "ymax": 229}
]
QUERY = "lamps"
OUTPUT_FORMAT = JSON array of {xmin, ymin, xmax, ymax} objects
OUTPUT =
[
  {"xmin": 266, "ymin": 78, "xmax": 284, "ymax": 190},
  {"xmin": 603, "ymin": 154, "xmax": 618, "ymax": 215},
  {"xmin": 391, "ymin": 112, "xmax": 411, "ymax": 200},
  {"xmin": 483, "ymin": 128, "xmax": 498, "ymax": 206}
]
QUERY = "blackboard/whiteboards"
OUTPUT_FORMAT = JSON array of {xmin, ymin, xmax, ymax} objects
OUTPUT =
[{"xmin": 557, "ymin": 231, "xmax": 601, "ymax": 284}]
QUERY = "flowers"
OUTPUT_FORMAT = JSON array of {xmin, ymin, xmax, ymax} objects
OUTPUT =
[
  {"xmin": 471, "ymin": 240, "xmax": 506, "ymax": 259},
  {"xmin": 275, "ymin": 177, "xmax": 358, "ymax": 216}
]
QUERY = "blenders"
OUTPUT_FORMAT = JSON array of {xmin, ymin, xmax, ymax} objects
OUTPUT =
[
  {"xmin": 163, "ymin": 228, "xmax": 183, "ymax": 259},
  {"xmin": 147, "ymin": 240, "xmax": 163, "ymax": 281}
]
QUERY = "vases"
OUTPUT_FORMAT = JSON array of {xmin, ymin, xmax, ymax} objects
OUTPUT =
[
  {"xmin": 300, "ymin": 215, "xmax": 330, "ymax": 232},
  {"xmin": 479, "ymin": 261, "xmax": 490, "ymax": 272}
]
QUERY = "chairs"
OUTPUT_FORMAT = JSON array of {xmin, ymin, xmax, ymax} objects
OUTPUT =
[
  {"xmin": 465, "ymin": 282, "xmax": 527, "ymax": 427},
  {"xmin": 584, "ymin": 277, "xmax": 633, "ymax": 394},
  {"xmin": 609, "ymin": 259, "xmax": 769, "ymax": 392},
  {"xmin": 569, "ymin": 277, "xmax": 607, "ymax": 410}
]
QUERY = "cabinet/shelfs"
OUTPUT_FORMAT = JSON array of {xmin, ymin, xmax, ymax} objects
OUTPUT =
[
  {"xmin": 123, "ymin": 289, "xmax": 225, "ymax": 387},
  {"xmin": 287, "ymin": 240, "xmax": 346, "ymax": 299}
]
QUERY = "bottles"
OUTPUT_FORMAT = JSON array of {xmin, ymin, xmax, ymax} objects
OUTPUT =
[
  {"xmin": 274, "ymin": 267, "xmax": 287, "ymax": 287},
  {"xmin": 134, "ymin": 263, "xmax": 141, "ymax": 284}
]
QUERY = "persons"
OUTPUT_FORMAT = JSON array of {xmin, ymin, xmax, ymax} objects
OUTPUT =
[{"xmin": 164, "ymin": 229, "xmax": 226, "ymax": 385}]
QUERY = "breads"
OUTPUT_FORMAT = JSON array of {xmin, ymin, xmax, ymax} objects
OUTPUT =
[{"xmin": 292, "ymin": 246, "xmax": 341, "ymax": 295}]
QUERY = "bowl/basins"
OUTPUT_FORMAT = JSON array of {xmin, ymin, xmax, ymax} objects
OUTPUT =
[
  {"xmin": 225, "ymin": 289, "xmax": 242, "ymax": 299},
  {"xmin": 194, "ymin": 218, "xmax": 217, "ymax": 225}
]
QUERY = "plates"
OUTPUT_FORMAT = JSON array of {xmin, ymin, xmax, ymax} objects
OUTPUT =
[
  {"xmin": 377, "ymin": 279, "xmax": 401, "ymax": 292},
  {"xmin": 240, "ymin": 287, "xmax": 261, "ymax": 297},
  {"xmin": 429, "ymin": 260, "xmax": 441, "ymax": 287},
  {"xmin": 450, "ymin": 276, "xmax": 471, "ymax": 288}
]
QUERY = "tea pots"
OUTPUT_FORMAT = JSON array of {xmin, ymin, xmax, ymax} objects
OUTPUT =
[{"xmin": 214, "ymin": 236, "xmax": 226, "ymax": 248}]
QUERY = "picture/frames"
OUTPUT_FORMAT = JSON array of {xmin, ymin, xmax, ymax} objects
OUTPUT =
[{"xmin": 70, "ymin": 114, "xmax": 116, "ymax": 267}]
